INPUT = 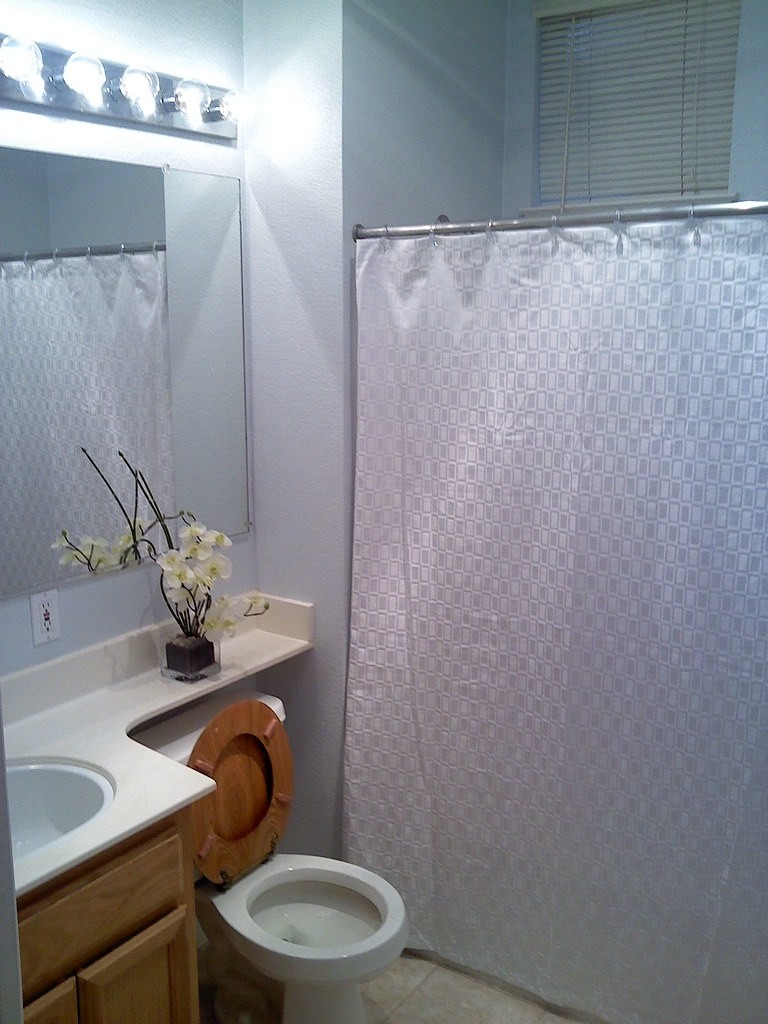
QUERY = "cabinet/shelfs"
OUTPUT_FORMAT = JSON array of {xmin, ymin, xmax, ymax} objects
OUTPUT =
[{"xmin": 16, "ymin": 804, "xmax": 198, "ymax": 1024}]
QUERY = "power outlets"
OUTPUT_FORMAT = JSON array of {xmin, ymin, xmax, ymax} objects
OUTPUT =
[{"xmin": 32, "ymin": 588, "xmax": 65, "ymax": 647}]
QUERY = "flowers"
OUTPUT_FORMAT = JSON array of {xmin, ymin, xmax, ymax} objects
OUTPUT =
[
  {"xmin": 115, "ymin": 451, "xmax": 273, "ymax": 639},
  {"xmin": 52, "ymin": 430, "xmax": 186, "ymax": 575}
]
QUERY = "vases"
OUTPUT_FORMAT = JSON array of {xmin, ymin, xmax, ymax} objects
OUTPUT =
[{"xmin": 159, "ymin": 631, "xmax": 223, "ymax": 686}]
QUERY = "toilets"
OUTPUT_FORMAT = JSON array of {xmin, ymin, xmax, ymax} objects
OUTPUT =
[{"xmin": 131, "ymin": 688, "xmax": 411, "ymax": 1023}]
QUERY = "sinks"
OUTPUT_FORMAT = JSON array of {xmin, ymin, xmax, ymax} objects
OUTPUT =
[{"xmin": 6, "ymin": 757, "xmax": 118, "ymax": 865}]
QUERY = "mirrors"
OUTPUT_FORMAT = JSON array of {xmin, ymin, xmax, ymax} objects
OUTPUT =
[{"xmin": 0, "ymin": 144, "xmax": 258, "ymax": 601}]
